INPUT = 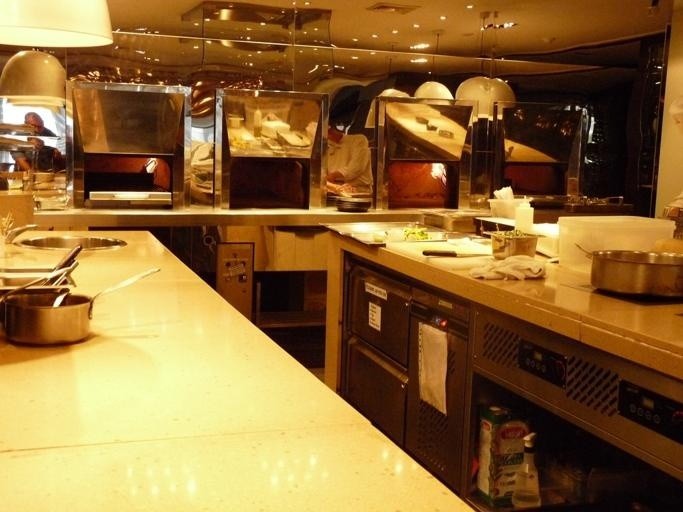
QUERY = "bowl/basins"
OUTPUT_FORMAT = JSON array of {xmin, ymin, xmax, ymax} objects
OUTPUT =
[
  {"xmin": 335, "ymin": 196, "xmax": 372, "ymax": 213},
  {"xmin": 30, "ymin": 171, "xmax": 66, "ymax": 190}
]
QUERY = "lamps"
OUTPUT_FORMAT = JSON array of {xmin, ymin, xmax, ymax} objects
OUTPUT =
[
  {"xmin": 375, "ymin": 12, "xmax": 515, "ymax": 116},
  {"xmin": 0, "ymin": 1, "xmax": 114, "ymax": 103}
]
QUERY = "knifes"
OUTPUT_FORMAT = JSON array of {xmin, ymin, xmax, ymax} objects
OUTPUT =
[{"xmin": 424, "ymin": 247, "xmax": 493, "ymax": 258}]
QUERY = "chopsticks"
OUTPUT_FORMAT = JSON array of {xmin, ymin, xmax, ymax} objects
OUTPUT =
[{"xmin": 50, "ymin": 272, "xmax": 68, "ymax": 286}]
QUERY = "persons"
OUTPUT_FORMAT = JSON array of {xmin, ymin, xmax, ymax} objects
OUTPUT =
[
  {"xmin": 10, "ymin": 137, "xmax": 64, "ymax": 173},
  {"xmin": 11, "ymin": 112, "xmax": 56, "ymax": 137},
  {"xmin": 325, "ymin": 124, "xmax": 373, "ymax": 195}
]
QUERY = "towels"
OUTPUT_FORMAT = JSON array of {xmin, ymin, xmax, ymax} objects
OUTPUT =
[{"xmin": 470, "ymin": 252, "xmax": 548, "ymax": 285}]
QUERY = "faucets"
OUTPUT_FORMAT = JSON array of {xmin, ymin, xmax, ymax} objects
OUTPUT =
[{"xmin": 4, "ymin": 224, "xmax": 40, "ymax": 244}]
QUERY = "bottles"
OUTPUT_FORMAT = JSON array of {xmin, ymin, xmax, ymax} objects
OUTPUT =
[{"xmin": 513, "ymin": 193, "xmax": 535, "ymax": 234}]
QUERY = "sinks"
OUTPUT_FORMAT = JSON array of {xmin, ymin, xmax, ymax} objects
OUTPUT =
[{"xmin": 15, "ymin": 236, "xmax": 127, "ymax": 251}]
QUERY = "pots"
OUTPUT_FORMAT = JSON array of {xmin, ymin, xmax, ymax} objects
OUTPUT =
[
  {"xmin": 589, "ymin": 247, "xmax": 682, "ymax": 298},
  {"xmin": 3, "ymin": 263, "xmax": 163, "ymax": 346}
]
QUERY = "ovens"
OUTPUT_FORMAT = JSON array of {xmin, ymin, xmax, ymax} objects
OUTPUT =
[{"xmin": 345, "ymin": 265, "xmax": 469, "ymax": 498}]
